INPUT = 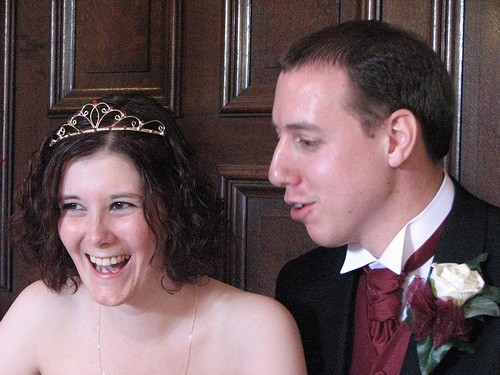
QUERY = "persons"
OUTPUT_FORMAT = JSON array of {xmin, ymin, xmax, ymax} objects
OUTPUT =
[
  {"xmin": 268, "ymin": 20, "xmax": 500, "ymax": 375},
  {"xmin": 0, "ymin": 90, "xmax": 308, "ymax": 375}
]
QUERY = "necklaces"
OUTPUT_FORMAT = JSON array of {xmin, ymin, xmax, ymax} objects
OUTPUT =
[{"xmin": 98, "ymin": 279, "xmax": 199, "ymax": 375}]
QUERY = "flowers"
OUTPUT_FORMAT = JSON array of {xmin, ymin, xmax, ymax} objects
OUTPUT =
[{"xmin": 405, "ymin": 262, "xmax": 500, "ymax": 375}]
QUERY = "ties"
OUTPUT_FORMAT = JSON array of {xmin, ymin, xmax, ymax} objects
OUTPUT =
[{"xmin": 363, "ymin": 213, "xmax": 451, "ymax": 356}]
